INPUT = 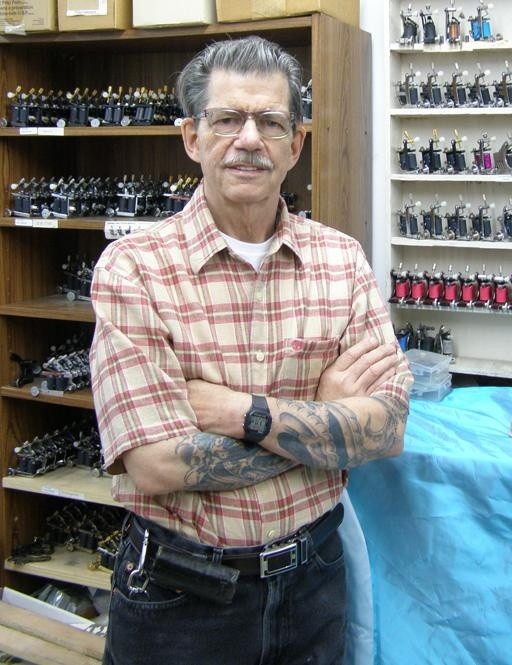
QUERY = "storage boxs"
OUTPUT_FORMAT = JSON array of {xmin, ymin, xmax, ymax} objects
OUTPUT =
[
  {"xmin": 0, "ymin": 0, "xmax": 56, "ymax": 34},
  {"xmin": 56, "ymin": 0, "xmax": 131, "ymax": 32},
  {"xmin": 214, "ymin": 0, "xmax": 359, "ymax": 29},
  {"xmin": 133, "ymin": 0, "xmax": 215, "ymax": 29}
]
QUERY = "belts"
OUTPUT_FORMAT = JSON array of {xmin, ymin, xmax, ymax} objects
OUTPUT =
[{"xmin": 125, "ymin": 502, "xmax": 345, "ymax": 579}]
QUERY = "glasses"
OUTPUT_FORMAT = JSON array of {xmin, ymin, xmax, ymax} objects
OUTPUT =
[{"xmin": 190, "ymin": 107, "xmax": 300, "ymax": 139}]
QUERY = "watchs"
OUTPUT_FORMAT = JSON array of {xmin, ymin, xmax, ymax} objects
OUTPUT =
[{"xmin": 242, "ymin": 392, "xmax": 272, "ymax": 447}]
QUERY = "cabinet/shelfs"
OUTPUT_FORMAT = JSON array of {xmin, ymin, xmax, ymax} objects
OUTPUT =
[
  {"xmin": 384, "ymin": 0, "xmax": 512, "ymax": 379},
  {"xmin": 0, "ymin": 14, "xmax": 373, "ymax": 665}
]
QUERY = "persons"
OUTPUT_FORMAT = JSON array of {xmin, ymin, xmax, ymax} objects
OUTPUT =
[{"xmin": 89, "ymin": 35, "xmax": 415, "ymax": 665}]
